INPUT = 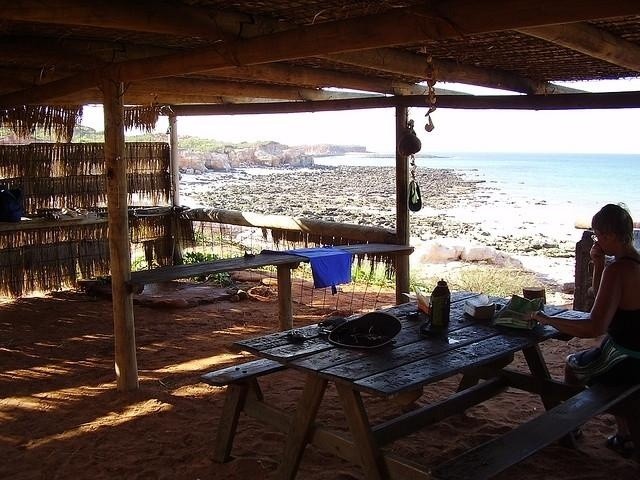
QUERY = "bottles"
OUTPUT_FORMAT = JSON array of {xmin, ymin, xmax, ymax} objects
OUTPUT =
[{"xmin": 431, "ymin": 280, "xmax": 450, "ymax": 328}]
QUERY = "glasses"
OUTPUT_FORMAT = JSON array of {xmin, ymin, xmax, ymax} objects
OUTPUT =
[{"xmin": 591, "ymin": 234, "xmax": 602, "ymax": 242}]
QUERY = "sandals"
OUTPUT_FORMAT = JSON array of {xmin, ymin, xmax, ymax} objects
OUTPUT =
[{"xmin": 606, "ymin": 435, "xmax": 633, "ymax": 459}]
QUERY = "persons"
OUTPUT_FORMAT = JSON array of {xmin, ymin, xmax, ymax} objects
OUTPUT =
[{"xmin": 523, "ymin": 203, "xmax": 640, "ymax": 458}]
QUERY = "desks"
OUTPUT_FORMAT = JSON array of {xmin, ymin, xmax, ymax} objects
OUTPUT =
[
  {"xmin": 229, "ymin": 289, "xmax": 595, "ymax": 480},
  {"xmin": 132, "ymin": 243, "xmax": 415, "ymax": 332}
]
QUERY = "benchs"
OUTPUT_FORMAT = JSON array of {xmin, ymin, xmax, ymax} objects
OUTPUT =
[
  {"xmin": 198, "ymin": 353, "xmax": 291, "ymax": 461},
  {"xmin": 426, "ymin": 382, "xmax": 640, "ymax": 479}
]
